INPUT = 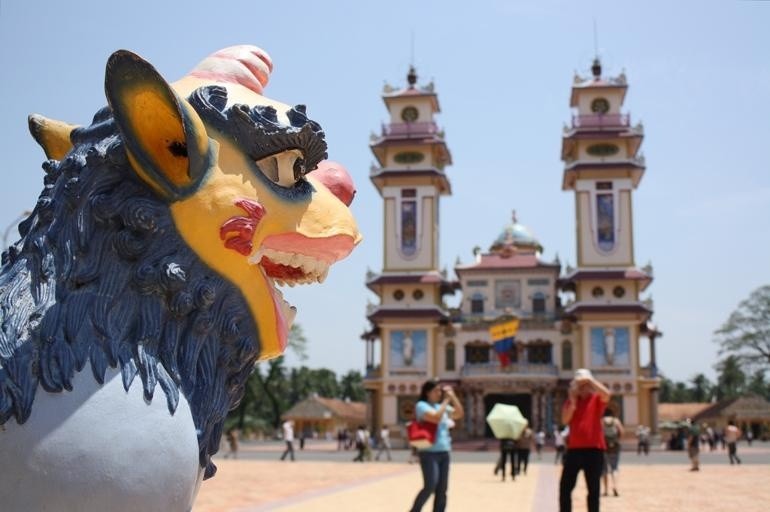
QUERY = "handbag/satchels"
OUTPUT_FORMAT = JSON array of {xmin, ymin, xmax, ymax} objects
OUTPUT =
[{"xmin": 405, "ymin": 401, "xmax": 437, "ymax": 449}]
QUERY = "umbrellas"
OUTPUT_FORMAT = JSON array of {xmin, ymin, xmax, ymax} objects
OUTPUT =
[{"xmin": 486, "ymin": 401, "xmax": 529, "ymax": 440}]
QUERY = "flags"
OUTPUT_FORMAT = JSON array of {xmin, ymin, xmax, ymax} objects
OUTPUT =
[{"xmin": 487, "ymin": 319, "xmax": 521, "ymax": 368}]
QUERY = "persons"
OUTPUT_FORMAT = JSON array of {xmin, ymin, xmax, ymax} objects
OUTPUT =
[
  {"xmin": 298, "ymin": 426, "xmax": 306, "ymax": 450},
  {"xmin": 552, "ymin": 422, "xmax": 567, "ymax": 466},
  {"xmin": 598, "ymin": 402, "xmax": 625, "ymax": 497},
  {"xmin": 498, "ymin": 437, "xmax": 518, "ymax": 482},
  {"xmin": 223, "ymin": 425, "xmax": 238, "ymax": 459},
  {"xmin": 558, "ymin": 368, "xmax": 611, "ymax": 511},
  {"xmin": 280, "ymin": 414, "xmax": 295, "ymax": 463},
  {"xmin": 337, "ymin": 422, "xmax": 394, "ymax": 462},
  {"xmin": 534, "ymin": 426, "xmax": 546, "ymax": 460},
  {"xmin": 407, "ymin": 377, "xmax": 465, "ymax": 512},
  {"xmin": 746, "ymin": 428, "xmax": 754, "ymax": 446},
  {"xmin": 517, "ymin": 419, "xmax": 536, "ymax": 473},
  {"xmin": 634, "ymin": 416, "xmax": 743, "ymax": 472}
]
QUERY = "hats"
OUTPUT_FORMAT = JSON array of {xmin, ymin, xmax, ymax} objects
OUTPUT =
[{"xmin": 570, "ymin": 368, "xmax": 593, "ymax": 382}]
